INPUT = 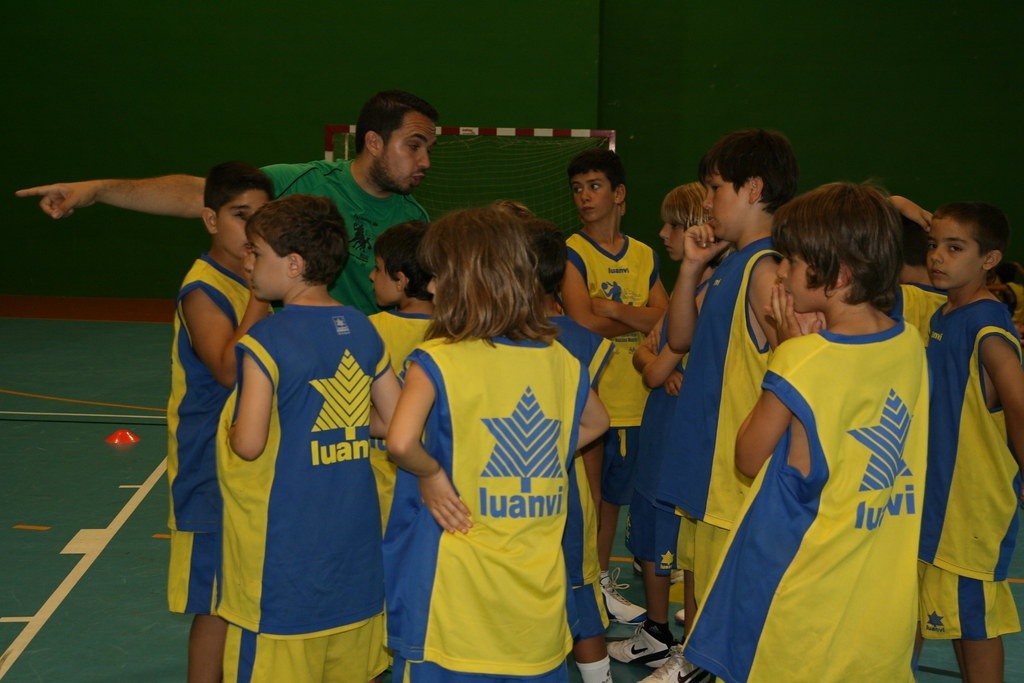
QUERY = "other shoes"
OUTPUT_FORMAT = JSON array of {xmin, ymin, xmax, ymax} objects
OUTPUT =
[
  {"xmin": 674, "ymin": 608, "xmax": 686, "ymax": 623},
  {"xmin": 634, "ymin": 559, "xmax": 643, "ymax": 575}
]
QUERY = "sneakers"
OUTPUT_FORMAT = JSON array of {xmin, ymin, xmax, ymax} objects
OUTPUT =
[
  {"xmin": 638, "ymin": 643, "xmax": 717, "ymax": 683},
  {"xmin": 599, "ymin": 567, "xmax": 648, "ymax": 625},
  {"xmin": 606, "ymin": 620, "xmax": 671, "ymax": 668}
]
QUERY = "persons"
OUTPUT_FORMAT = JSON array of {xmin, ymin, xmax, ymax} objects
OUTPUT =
[
  {"xmin": 165, "ymin": 163, "xmax": 274, "ymax": 683},
  {"xmin": 923, "ymin": 198, "xmax": 1024, "ymax": 683},
  {"xmin": 383, "ymin": 198, "xmax": 610, "ymax": 683},
  {"xmin": 562, "ymin": 146, "xmax": 671, "ymax": 622},
  {"xmin": 218, "ymin": 192, "xmax": 402, "ymax": 683},
  {"xmin": 14, "ymin": 88, "xmax": 442, "ymax": 318},
  {"xmin": 661, "ymin": 130, "xmax": 806, "ymax": 633},
  {"xmin": 358, "ymin": 218, "xmax": 438, "ymax": 539},
  {"xmin": 682, "ymin": 180, "xmax": 933, "ymax": 683},
  {"xmin": 514, "ymin": 182, "xmax": 1023, "ymax": 683}
]
